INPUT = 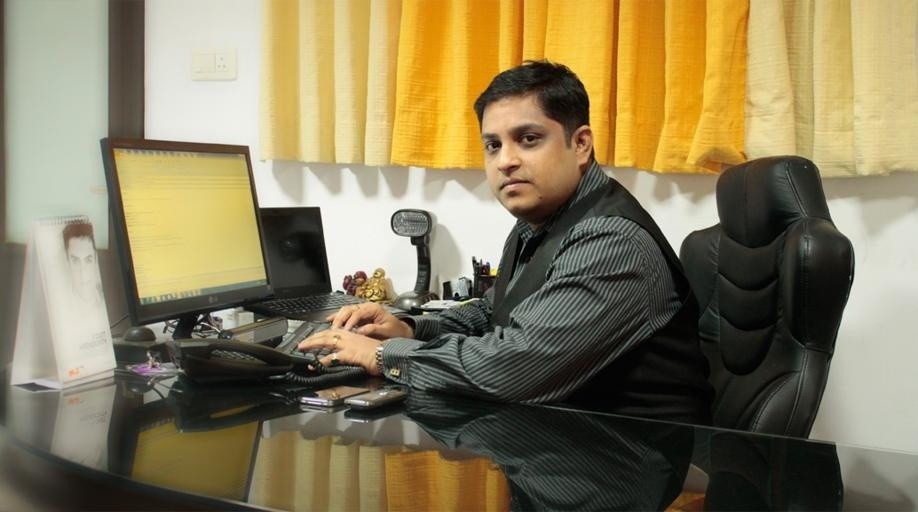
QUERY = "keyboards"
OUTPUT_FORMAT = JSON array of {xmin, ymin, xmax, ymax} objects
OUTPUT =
[{"xmin": 272, "ymin": 319, "xmax": 358, "ymax": 367}]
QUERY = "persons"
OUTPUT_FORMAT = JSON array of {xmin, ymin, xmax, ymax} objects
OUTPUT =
[
  {"xmin": 389, "ymin": 383, "xmax": 699, "ymax": 508},
  {"xmin": 61, "ymin": 223, "xmax": 100, "ymax": 301},
  {"xmin": 294, "ymin": 58, "xmax": 703, "ymax": 510},
  {"xmin": 356, "ymin": 267, "xmax": 387, "ymax": 301}
]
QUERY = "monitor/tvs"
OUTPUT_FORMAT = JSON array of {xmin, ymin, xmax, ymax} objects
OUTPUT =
[{"xmin": 100, "ymin": 138, "xmax": 275, "ymax": 343}]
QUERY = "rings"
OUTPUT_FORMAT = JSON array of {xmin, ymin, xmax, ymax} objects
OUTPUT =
[
  {"xmin": 330, "ymin": 351, "xmax": 339, "ymax": 365},
  {"xmin": 332, "ymin": 333, "xmax": 341, "ymax": 349}
]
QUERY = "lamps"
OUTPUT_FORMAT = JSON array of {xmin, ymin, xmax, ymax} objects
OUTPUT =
[{"xmin": 389, "ymin": 208, "xmax": 441, "ymax": 311}]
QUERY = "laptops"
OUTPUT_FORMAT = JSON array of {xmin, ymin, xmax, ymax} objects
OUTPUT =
[{"xmin": 243, "ymin": 205, "xmax": 407, "ymax": 322}]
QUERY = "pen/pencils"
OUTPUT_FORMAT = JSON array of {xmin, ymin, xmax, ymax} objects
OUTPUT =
[
  {"xmin": 473, "ymin": 256, "xmax": 490, "ymax": 276},
  {"xmin": 468, "ymin": 280, "xmax": 472, "ymax": 298}
]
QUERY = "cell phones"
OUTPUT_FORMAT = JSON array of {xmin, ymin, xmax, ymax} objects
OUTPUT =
[
  {"xmin": 301, "ymin": 385, "xmax": 370, "ymax": 410},
  {"xmin": 343, "ymin": 387, "xmax": 407, "ymax": 411}
]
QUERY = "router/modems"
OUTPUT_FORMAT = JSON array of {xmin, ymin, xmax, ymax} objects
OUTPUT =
[{"xmin": 110, "ymin": 336, "xmax": 169, "ymax": 364}]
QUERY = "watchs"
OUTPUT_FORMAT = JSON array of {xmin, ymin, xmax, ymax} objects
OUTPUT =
[{"xmin": 375, "ymin": 338, "xmax": 392, "ymax": 377}]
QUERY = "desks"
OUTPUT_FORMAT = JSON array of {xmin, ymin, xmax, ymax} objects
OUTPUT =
[{"xmin": 2, "ymin": 310, "xmax": 866, "ymax": 510}]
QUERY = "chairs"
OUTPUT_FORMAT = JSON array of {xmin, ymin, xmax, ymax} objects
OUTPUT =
[{"xmin": 678, "ymin": 155, "xmax": 857, "ymax": 442}]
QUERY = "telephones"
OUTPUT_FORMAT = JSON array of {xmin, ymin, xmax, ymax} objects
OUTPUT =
[{"xmin": 174, "ymin": 338, "xmax": 294, "ymax": 387}]
